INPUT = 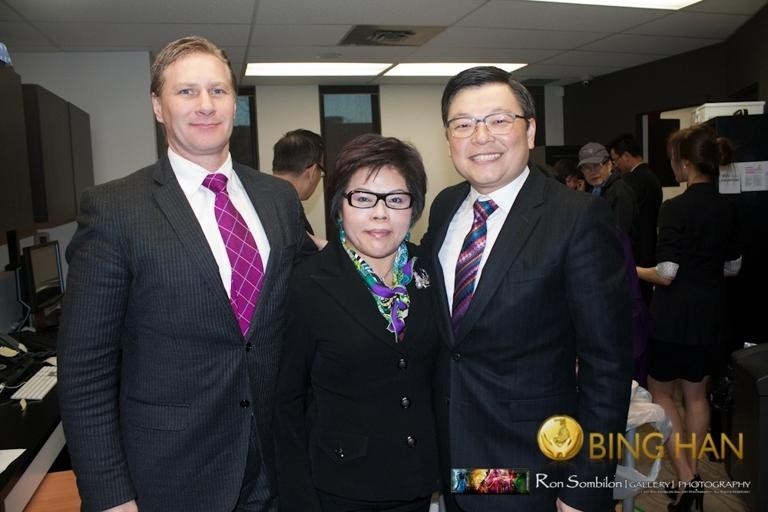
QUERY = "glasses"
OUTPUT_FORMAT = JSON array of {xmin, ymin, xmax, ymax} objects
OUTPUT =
[
  {"xmin": 446, "ymin": 112, "xmax": 528, "ymax": 139},
  {"xmin": 315, "ymin": 161, "xmax": 326, "ymax": 178},
  {"xmin": 342, "ymin": 189, "xmax": 414, "ymax": 211}
]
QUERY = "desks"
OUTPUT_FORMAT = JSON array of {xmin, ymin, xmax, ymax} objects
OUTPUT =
[
  {"xmin": 22, "ymin": 468, "xmax": 84, "ymax": 511},
  {"xmin": 0, "ymin": 307, "xmax": 68, "ymax": 512}
]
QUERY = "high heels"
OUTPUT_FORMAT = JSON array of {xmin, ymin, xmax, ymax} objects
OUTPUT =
[{"xmin": 664, "ymin": 473, "xmax": 704, "ymax": 512}]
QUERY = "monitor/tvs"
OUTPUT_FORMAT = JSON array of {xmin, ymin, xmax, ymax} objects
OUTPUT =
[{"xmin": 18, "ymin": 239, "xmax": 65, "ymax": 334}]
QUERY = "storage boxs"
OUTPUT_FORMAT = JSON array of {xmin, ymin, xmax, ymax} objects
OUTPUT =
[{"xmin": 688, "ymin": 98, "xmax": 764, "ymax": 128}]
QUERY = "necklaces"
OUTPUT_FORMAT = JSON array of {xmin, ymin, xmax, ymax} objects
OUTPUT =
[{"xmin": 380, "ymin": 267, "xmax": 392, "ymax": 282}]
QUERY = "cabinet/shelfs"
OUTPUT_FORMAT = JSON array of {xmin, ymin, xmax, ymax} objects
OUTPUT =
[
  {"xmin": 0, "ymin": 63, "xmax": 35, "ymax": 234},
  {"xmin": 693, "ymin": 113, "xmax": 767, "ymax": 377},
  {"xmin": 21, "ymin": 83, "xmax": 96, "ymax": 227}
]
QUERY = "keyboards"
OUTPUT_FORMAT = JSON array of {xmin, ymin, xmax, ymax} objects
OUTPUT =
[{"xmin": 10, "ymin": 366, "xmax": 58, "ymax": 401}]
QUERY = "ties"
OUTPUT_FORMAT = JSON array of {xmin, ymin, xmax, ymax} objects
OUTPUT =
[
  {"xmin": 201, "ymin": 173, "xmax": 264, "ymax": 341},
  {"xmin": 452, "ymin": 199, "xmax": 500, "ymax": 332}
]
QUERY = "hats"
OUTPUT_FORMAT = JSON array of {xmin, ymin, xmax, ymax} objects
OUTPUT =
[{"xmin": 576, "ymin": 142, "xmax": 610, "ymax": 168}]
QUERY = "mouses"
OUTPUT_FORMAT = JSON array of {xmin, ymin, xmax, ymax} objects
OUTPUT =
[{"xmin": 41, "ymin": 356, "xmax": 57, "ymax": 366}]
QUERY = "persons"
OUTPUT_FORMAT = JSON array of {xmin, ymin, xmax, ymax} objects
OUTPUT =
[
  {"xmin": 278, "ymin": 137, "xmax": 435, "ymax": 511},
  {"xmin": 272, "ymin": 129, "xmax": 327, "ymax": 251},
  {"xmin": 419, "ymin": 66, "xmax": 634, "ymax": 512},
  {"xmin": 58, "ymin": 33, "xmax": 318, "ymax": 512}
]
